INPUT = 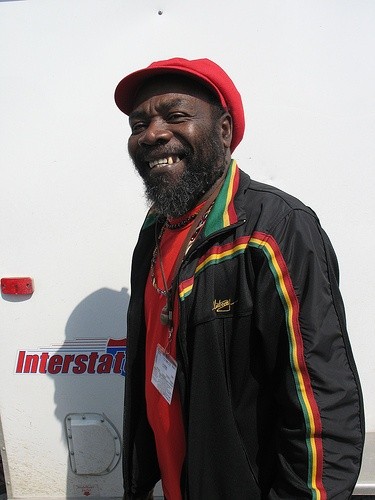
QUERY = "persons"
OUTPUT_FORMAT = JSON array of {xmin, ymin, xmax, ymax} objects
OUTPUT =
[{"xmin": 112, "ymin": 56, "xmax": 367, "ymax": 500}]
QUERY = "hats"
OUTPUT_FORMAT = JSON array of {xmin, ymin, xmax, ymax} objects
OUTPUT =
[{"xmin": 114, "ymin": 57, "xmax": 247, "ymax": 152}]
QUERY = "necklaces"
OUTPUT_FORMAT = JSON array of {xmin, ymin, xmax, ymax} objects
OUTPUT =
[
  {"xmin": 149, "ymin": 200, "xmax": 217, "ymax": 325},
  {"xmin": 165, "ymin": 207, "xmax": 200, "ymax": 231}
]
